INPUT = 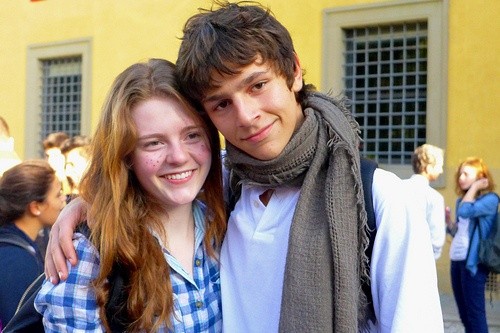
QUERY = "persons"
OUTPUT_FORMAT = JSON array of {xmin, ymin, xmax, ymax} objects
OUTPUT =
[
  {"xmin": 0, "ymin": 2, "xmax": 448, "ymax": 331},
  {"xmin": 442, "ymin": 157, "xmax": 499, "ymax": 333}
]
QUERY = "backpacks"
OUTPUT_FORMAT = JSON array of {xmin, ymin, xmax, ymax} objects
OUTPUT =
[{"xmin": 477, "ymin": 192, "xmax": 500, "ymax": 274}]
